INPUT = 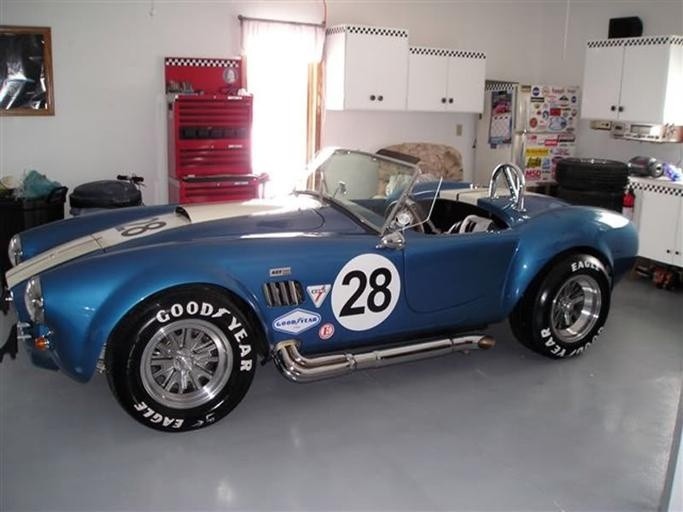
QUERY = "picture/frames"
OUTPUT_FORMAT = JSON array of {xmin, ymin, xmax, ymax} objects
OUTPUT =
[{"xmin": 0, "ymin": 24, "xmax": 56, "ymax": 117}]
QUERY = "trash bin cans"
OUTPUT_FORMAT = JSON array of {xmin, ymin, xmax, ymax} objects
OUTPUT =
[
  {"xmin": 69, "ymin": 175, "xmax": 144, "ymax": 217},
  {"xmin": 0, "ymin": 187, "xmax": 66, "ymax": 285}
]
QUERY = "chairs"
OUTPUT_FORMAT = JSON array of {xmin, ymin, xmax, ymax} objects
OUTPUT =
[
  {"xmin": 374, "ymin": 143, "xmax": 464, "ymax": 196},
  {"xmin": 445, "ymin": 215, "xmax": 499, "ymax": 235}
]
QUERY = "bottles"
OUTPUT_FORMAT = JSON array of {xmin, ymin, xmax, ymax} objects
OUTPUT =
[{"xmin": 662, "ymin": 161, "xmax": 681, "ymax": 181}]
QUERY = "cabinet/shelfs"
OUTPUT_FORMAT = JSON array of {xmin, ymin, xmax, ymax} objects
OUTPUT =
[
  {"xmin": 627, "ymin": 175, "xmax": 683, "ymax": 268},
  {"xmin": 323, "ymin": 24, "xmax": 486, "ymax": 114},
  {"xmin": 579, "ymin": 35, "xmax": 683, "ymax": 124},
  {"xmin": 163, "ymin": 56, "xmax": 267, "ymax": 203}
]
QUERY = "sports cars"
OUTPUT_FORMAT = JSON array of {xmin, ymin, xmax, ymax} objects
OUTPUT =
[{"xmin": 4, "ymin": 149, "xmax": 639, "ymax": 433}]
